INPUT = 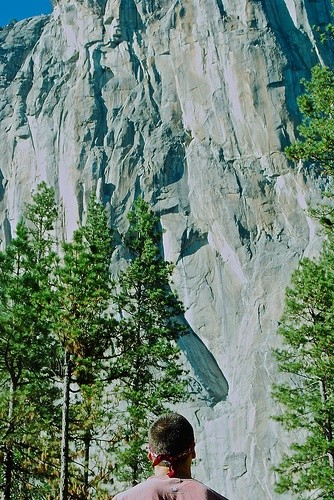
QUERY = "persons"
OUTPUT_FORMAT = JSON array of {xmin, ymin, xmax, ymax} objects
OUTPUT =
[{"xmin": 110, "ymin": 413, "xmax": 231, "ymax": 500}]
